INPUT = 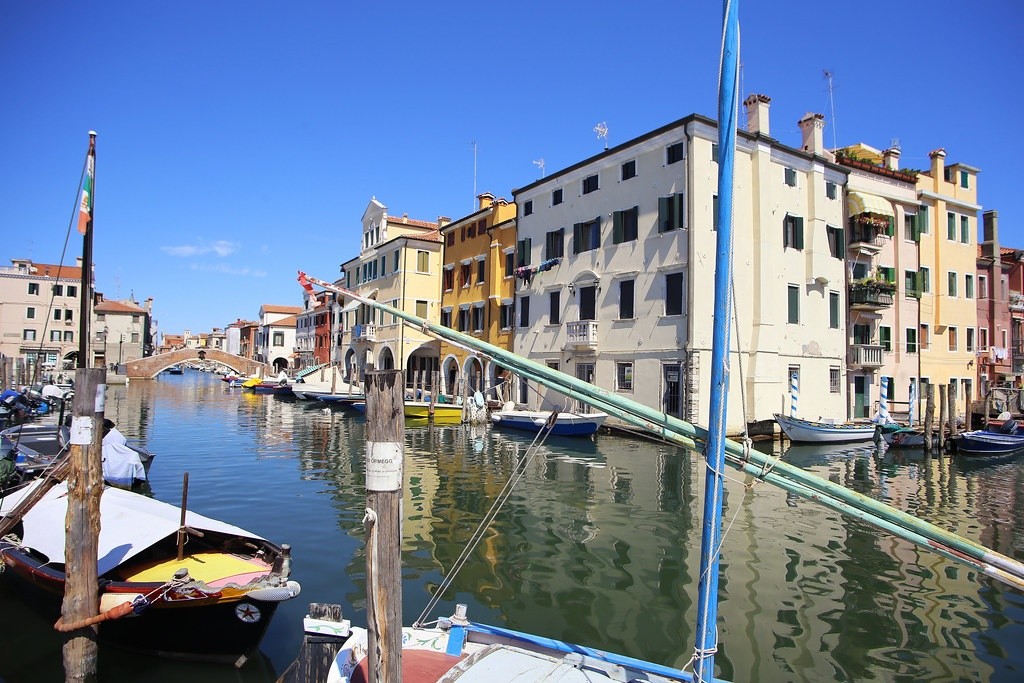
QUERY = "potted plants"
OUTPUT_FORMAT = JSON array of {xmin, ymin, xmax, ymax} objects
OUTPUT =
[{"xmin": 862, "ymin": 277, "xmax": 897, "ymax": 290}]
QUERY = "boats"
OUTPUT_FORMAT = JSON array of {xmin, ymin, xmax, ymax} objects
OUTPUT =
[
  {"xmin": 490, "ymin": 428, "xmax": 607, "ymax": 469},
  {"xmin": 327, "ymin": 601, "xmax": 730, "ymax": 683},
  {"xmin": 781, "ymin": 441, "xmax": 878, "ymax": 467},
  {"xmin": 164, "ymin": 361, "xmax": 464, "ymax": 417},
  {"xmin": 491, "ymin": 411, "xmax": 608, "ymax": 436},
  {"xmin": 772, "ymin": 387, "xmax": 1024, "ymax": 458},
  {"xmin": 0, "ymin": 384, "xmax": 301, "ymax": 654}
]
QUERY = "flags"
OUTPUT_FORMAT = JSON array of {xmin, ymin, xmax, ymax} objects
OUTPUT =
[{"xmin": 75, "ymin": 146, "xmax": 95, "ymax": 236}]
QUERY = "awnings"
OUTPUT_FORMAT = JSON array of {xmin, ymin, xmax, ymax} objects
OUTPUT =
[
  {"xmin": 339, "ymin": 289, "xmax": 378, "ymax": 314},
  {"xmin": 848, "ymin": 191, "xmax": 895, "ymax": 218},
  {"xmin": 828, "ymin": 142, "xmax": 885, "ymax": 166}
]
